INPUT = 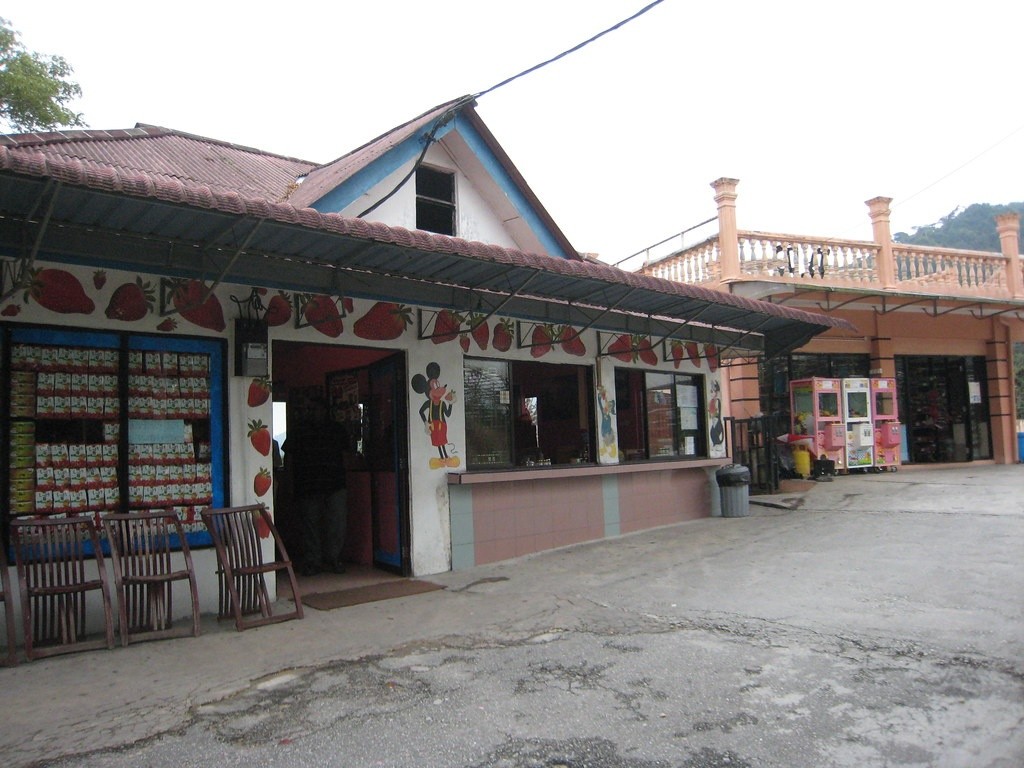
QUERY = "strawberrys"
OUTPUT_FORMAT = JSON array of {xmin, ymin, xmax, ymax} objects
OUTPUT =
[
  {"xmin": 18, "ymin": 262, "xmax": 587, "ymax": 359},
  {"xmin": 247, "ymin": 374, "xmax": 272, "ymax": 538},
  {"xmin": 606, "ymin": 336, "xmax": 719, "ymax": 372}
]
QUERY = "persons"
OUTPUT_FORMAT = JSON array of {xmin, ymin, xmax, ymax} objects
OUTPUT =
[{"xmin": 273, "ymin": 395, "xmax": 353, "ymax": 577}]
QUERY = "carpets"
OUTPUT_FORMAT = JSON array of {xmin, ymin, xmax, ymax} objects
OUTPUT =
[{"xmin": 288, "ymin": 579, "xmax": 447, "ymax": 611}]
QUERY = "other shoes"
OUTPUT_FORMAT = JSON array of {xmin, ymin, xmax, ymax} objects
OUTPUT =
[
  {"xmin": 326, "ymin": 558, "xmax": 346, "ymax": 573},
  {"xmin": 302, "ymin": 562, "xmax": 320, "ymax": 575}
]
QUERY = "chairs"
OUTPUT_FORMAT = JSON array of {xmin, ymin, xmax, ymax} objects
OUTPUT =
[
  {"xmin": 11, "ymin": 516, "xmax": 115, "ymax": 663},
  {"xmin": 102, "ymin": 511, "xmax": 200, "ymax": 648},
  {"xmin": 0, "ymin": 542, "xmax": 17, "ymax": 669},
  {"xmin": 200, "ymin": 502, "xmax": 303, "ymax": 633}
]
